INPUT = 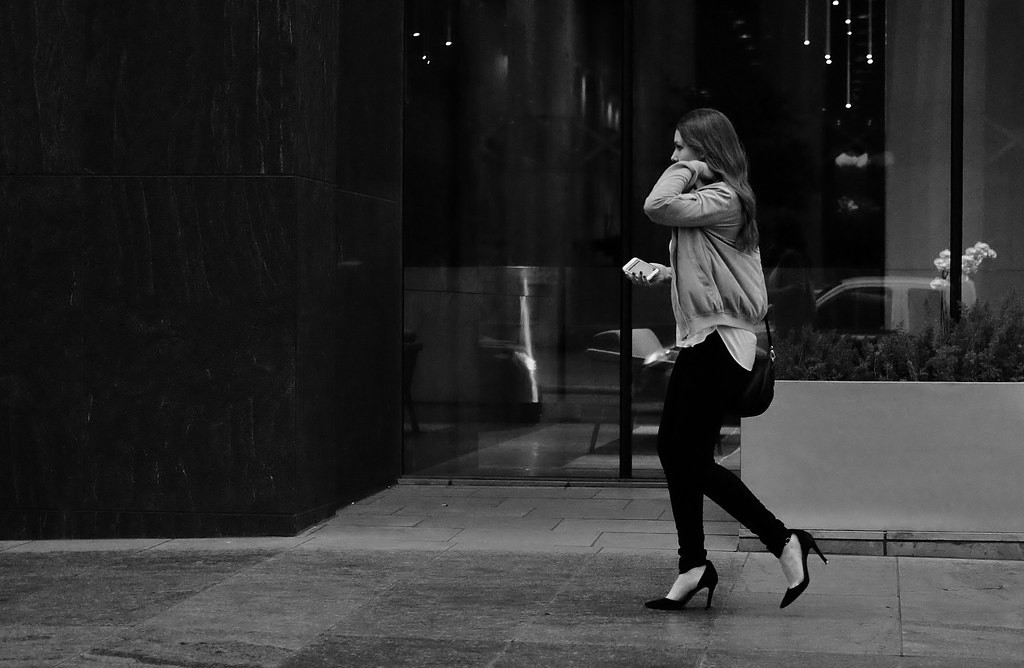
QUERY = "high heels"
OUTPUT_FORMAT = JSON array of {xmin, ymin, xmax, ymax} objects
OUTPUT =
[
  {"xmin": 779, "ymin": 529, "xmax": 829, "ymax": 609},
  {"xmin": 644, "ymin": 560, "xmax": 718, "ymax": 611}
]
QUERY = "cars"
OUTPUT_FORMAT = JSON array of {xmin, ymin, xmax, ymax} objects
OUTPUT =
[{"xmin": 755, "ymin": 279, "xmax": 951, "ymax": 340}]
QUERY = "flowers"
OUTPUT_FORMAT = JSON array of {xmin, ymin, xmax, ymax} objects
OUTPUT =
[{"xmin": 930, "ymin": 241, "xmax": 998, "ymax": 334}]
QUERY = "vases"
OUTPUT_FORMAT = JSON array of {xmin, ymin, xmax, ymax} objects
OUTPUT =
[{"xmin": 944, "ymin": 272, "xmax": 976, "ymax": 321}]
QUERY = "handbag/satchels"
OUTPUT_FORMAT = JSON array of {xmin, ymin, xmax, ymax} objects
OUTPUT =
[{"xmin": 726, "ymin": 316, "xmax": 777, "ymax": 420}]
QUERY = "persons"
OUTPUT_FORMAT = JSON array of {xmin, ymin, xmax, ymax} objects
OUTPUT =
[{"xmin": 624, "ymin": 108, "xmax": 829, "ymax": 611}]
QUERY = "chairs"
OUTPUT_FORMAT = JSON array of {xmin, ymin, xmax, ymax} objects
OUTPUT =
[{"xmin": 581, "ymin": 326, "xmax": 724, "ymax": 457}]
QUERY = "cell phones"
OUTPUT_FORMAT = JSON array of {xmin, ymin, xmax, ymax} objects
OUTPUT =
[{"xmin": 621, "ymin": 257, "xmax": 660, "ymax": 282}]
